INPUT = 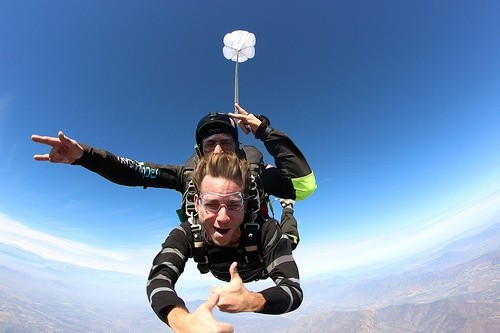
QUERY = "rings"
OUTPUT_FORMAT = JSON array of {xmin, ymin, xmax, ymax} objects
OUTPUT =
[{"xmin": 247, "ymin": 114, "xmax": 249, "ymax": 115}]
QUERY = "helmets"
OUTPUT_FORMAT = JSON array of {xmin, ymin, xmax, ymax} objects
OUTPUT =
[{"xmin": 194, "ymin": 110, "xmax": 238, "ymax": 142}]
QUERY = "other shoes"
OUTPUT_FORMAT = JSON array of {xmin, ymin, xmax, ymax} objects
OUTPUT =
[{"xmin": 279, "ymin": 197, "xmax": 296, "ymax": 207}]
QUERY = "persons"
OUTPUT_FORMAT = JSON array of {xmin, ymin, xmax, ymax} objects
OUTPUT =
[
  {"xmin": 146, "ymin": 150, "xmax": 304, "ymax": 332},
  {"xmin": 30, "ymin": 103, "xmax": 317, "ymax": 224}
]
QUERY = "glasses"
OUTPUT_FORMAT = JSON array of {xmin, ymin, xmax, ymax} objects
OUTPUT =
[{"xmin": 198, "ymin": 191, "xmax": 245, "ymax": 212}]
